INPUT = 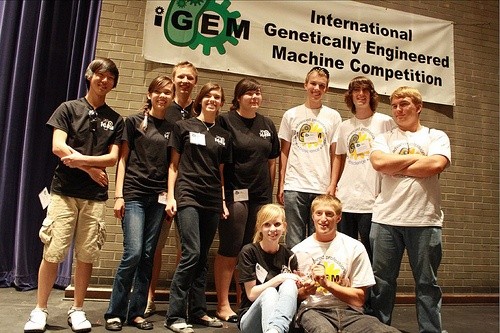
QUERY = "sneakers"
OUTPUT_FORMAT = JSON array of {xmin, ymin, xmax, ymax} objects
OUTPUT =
[
  {"xmin": 67, "ymin": 306, "xmax": 92, "ymax": 332},
  {"xmin": 23, "ymin": 308, "xmax": 48, "ymax": 332}
]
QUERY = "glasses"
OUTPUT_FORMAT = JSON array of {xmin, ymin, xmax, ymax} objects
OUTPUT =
[{"xmin": 308, "ymin": 67, "xmax": 329, "ymax": 80}]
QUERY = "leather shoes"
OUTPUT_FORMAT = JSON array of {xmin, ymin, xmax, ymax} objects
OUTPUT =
[
  {"xmin": 128, "ymin": 318, "xmax": 153, "ymax": 330},
  {"xmin": 105, "ymin": 319, "xmax": 122, "ymax": 330}
]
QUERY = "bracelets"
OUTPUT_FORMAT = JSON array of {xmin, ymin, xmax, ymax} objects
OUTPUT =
[
  {"xmin": 113, "ymin": 196, "xmax": 123, "ymax": 199},
  {"xmin": 222, "ymin": 198, "xmax": 225, "ymax": 200}
]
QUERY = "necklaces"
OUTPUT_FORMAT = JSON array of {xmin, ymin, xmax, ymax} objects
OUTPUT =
[{"xmin": 198, "ymin": 116, "xmax": 215, "ymax": 132}]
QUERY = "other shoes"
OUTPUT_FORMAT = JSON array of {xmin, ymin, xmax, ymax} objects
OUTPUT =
[
  {"xmin": 164, "ymin": 320, "xmax": 194, "ymax": 333},
  {"xmin": 144, "ymin": 306, "xmax": 156, "ymax": 318},
  {"xmin": 215, "ymin": 313, "xmax": 239, "ymax": 323},
  {"xmin": 190, "ymin": 317, "xmax": 223, "ymax": 327}
]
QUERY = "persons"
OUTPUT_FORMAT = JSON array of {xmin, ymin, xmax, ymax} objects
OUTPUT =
[
  {"xmin": 236, "ymin": 204, "xmax": 302, "ymax": 333},
  {"xmin": 326, "ymin": 75, "xmax": 394, "ymax": 316},
  {"xmin": 23, "ymin": 59, "xmax": 124, "ymax": 333},
  {"xmin": 164, "ymin": 82, "xmax": 232, "ymax": 333},
  {"xmin": 143, "ymin": 62, "xmax": 198, "ymax": 317},
  {"xmin": 290, "ymin": 195, "xmax": 401, "ymax": 333},
  {"xmin": 103, "ymin": 76, "xmax": 176, "ymax": 330},
  {"xmin": 212, "ymin": 78, "xmax": 280, "ymax": 324},
  {"xmin": 366, "ymin": 86, "xmax": 451, "ymax": 333},
  {"xmin": 278, "ymin": 66, "xmax": 342, "ymax": 249}
]
